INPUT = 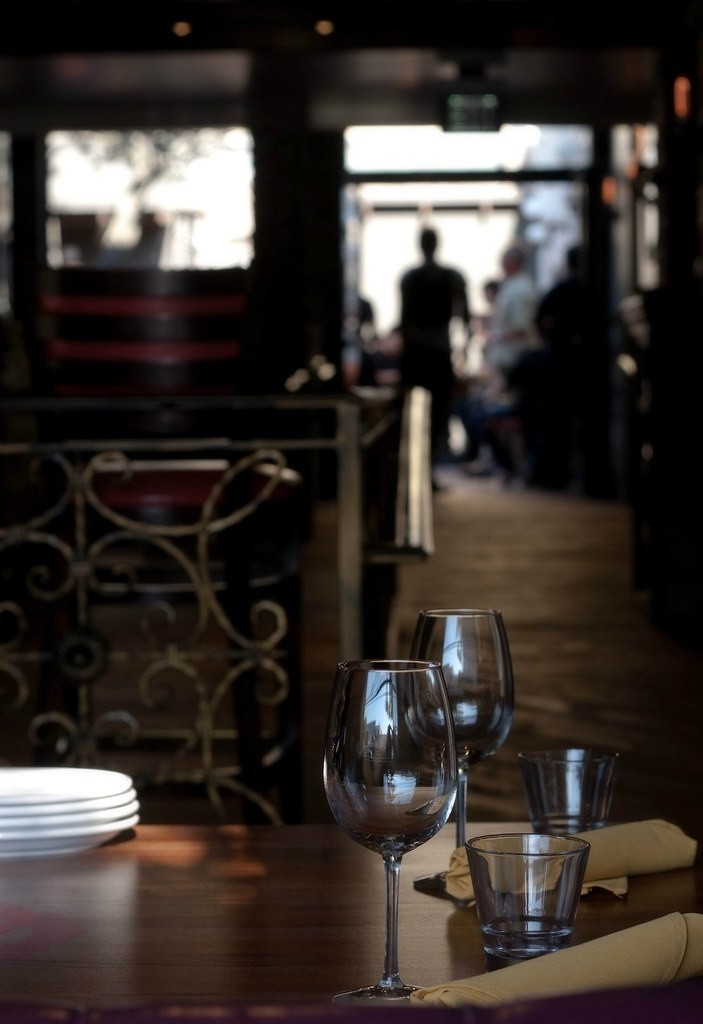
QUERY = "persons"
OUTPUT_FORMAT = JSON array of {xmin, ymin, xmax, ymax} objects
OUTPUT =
[{"xmin": 380, "ymin": 229, "xmax": 617, "ymax": 473}]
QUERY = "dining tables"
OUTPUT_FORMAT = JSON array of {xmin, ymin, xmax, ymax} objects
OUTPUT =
[{"xmin": 0, "ymin": 818, "xmax": 702, "ymax": 1024}]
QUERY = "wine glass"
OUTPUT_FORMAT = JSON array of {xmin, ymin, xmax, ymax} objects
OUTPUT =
[
  {"xmin": 412, "ymin": 608, "xmax": 512, "ymax": 886},
  {"xmin": 322, "ymin": 662, "xmax": 458, "ymax": 1003}
]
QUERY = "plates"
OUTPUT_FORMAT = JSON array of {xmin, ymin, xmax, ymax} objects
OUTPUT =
[{"xmin": 0, "ymin": 768, "xmax": 140, "ymax": 856}]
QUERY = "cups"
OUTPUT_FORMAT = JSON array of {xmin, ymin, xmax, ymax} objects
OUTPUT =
[
  {"xmin": 465, "ymin": 834, "xmax": 591, "ymax": 963},
  {"xmin": 517, "ymin": 748, "xmax": 618, "ymax": 834}
]
